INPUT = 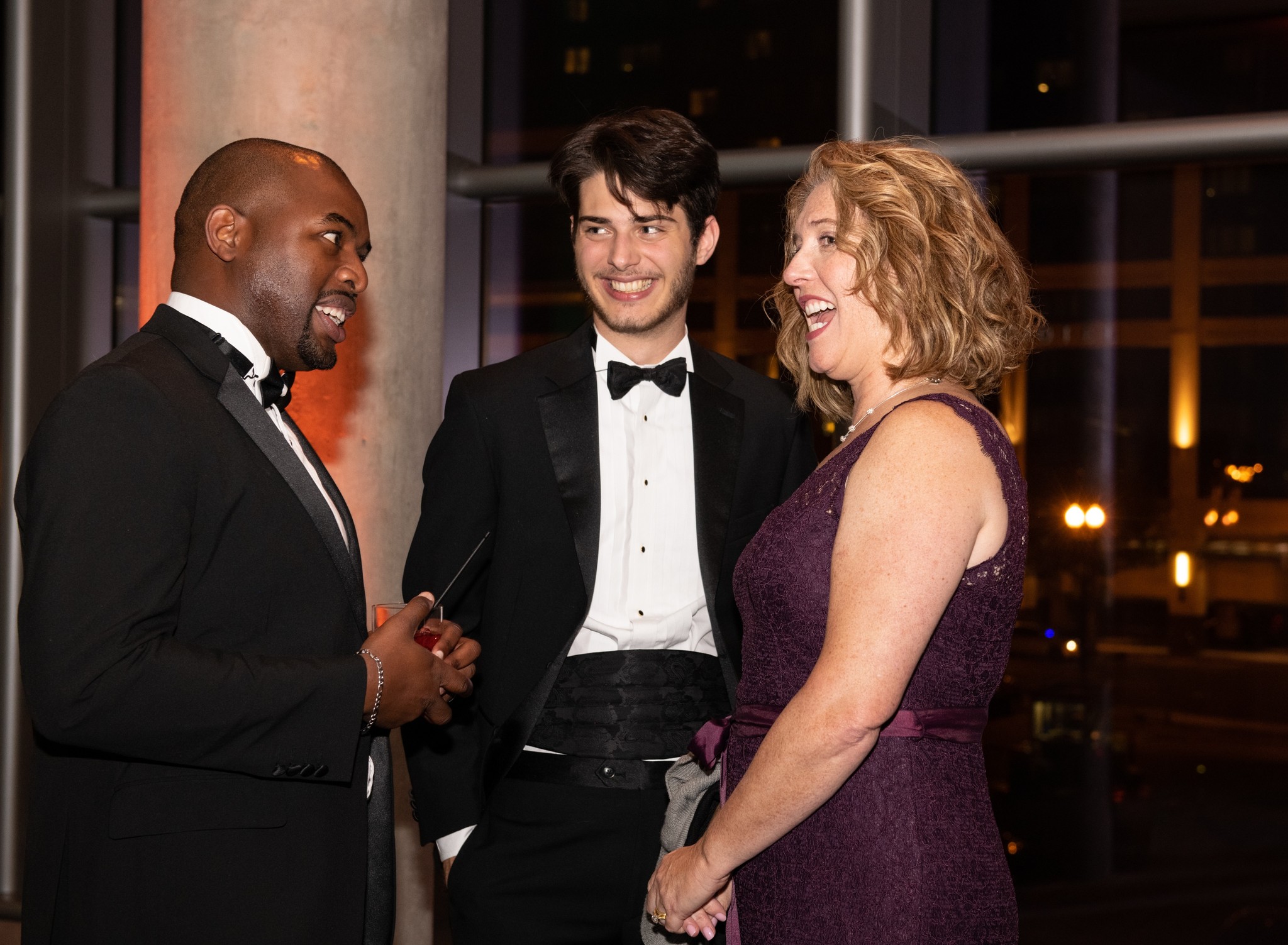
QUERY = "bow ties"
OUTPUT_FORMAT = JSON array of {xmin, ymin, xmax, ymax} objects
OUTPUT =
[
  {"xmin": 607, "ymin": 356, "xmax": 691, "ymax": 400},
  {"xmin": 192, "ymin": 322, "xmax": 295, "ymax": 413}
]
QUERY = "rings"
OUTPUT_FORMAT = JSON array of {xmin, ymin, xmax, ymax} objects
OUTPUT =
[{"xmin": 651, "ymin": 910, "xmax": 666, "ymax": 923}]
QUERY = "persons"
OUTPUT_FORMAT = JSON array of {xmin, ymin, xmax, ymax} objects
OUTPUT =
[
  {"xmin": 646, "ymin": 135, "xmax": 1041, "ymax": 945},
  {"xmin": 397, "ymin": 108, "xmax": 830, "ymax": 945},
  {"xmin": 22, "ymin": 138, "xmax": 479, "ymax": 945}
]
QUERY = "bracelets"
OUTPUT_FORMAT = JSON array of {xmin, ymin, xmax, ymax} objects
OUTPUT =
[{"xmin": 356, "ymin": 650, "xmax": 384, "ymax": 733}]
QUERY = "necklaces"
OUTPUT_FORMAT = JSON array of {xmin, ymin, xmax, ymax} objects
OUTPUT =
[{"xmin": 839, "ymin": 375, "xmax": 940, "ymax": 442}]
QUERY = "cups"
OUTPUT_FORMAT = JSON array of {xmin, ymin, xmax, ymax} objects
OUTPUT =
[{"xmin": 372, "ymin": 603, "xmax": 443, "ymax": 653}]
